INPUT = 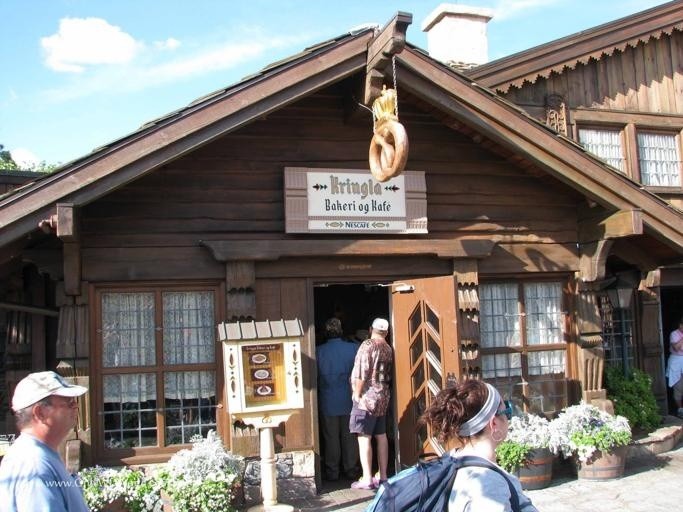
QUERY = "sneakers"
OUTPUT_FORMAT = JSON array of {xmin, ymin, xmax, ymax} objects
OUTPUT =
[
  {"xmin": 351, "ymin": 480, "xmax": 373, "ymax": 490},
  {"xmin": 359, "ymin": 476, "xmax": 380, "ymax": 485}
]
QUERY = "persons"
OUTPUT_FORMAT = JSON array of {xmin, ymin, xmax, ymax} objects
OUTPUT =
[
  {"xmin": 316, "ymin": 317, "xmax": 393, "ymax": 490},
  {"xmin": 665, "ymin": 317, "xmax": 683, "ymax": 420},
  {"xmin": 413, "ymin": 380, "xmax": 539, "ymax": 512},
  {"xmin": 0, "ymin": 370, "xmax": 90, "ymax": 512}
]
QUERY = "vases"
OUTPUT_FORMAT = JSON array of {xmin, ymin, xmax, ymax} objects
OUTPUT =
[{"xmin": 99, "ymin": 493, "xmax": 129, "ymax": 511}]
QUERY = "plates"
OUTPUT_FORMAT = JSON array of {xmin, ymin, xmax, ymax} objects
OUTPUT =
[
  {"xmin": 256, "ymin": 386, "xmax": 271, "ymax": 396},
  {"xmin": 251, "ymin": 354, "xmax": 266, "ymax": 364},
  {"xmin": 253, "ymin": 369, "xmax": 269, "ymax": 380}
]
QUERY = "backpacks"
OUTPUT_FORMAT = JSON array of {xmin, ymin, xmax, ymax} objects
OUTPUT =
[{"xmin": 363, "ymin": 451, "xmax": 540, "ymax": 512}]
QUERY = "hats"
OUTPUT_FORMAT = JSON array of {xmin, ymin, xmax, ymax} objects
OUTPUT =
[
  {"xmin": 349, "ymin": 330, "xmax": 368, "ymax": 342},
  {"xmin": 12, "ymin": 371, "xmax": 90, "ymax": 411},
  {"xmin": 372, "ymin": 317, "xmax": 389, "ymax": 330},
  {"xmin": 325, "ymin": 318, "xmax": 343, "ymax": 333}
]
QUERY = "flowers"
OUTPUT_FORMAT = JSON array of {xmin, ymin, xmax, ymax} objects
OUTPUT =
[
  {"xmin": 493, "ymin": 412, "xmax": 555, "ymax": 472},
  {"xmin": 548, "ymin": 403, "xmax": 634, "ymax": 463},
  {"xmin": 74, "ymin": 462, "xmax": 148, "ymax": 512},
  {"xmin": 142, "ymin": 429, "xmax": 248, "ymax": 511}
]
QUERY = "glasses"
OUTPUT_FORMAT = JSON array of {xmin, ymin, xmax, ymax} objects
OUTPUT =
[{"xmin": 498, "ymin": 399, "xmax": 512, "ymax": 419}]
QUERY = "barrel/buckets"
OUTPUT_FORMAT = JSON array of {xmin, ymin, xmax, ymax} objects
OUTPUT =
[{"xmin": 99, "ymin": 495, "xmax": 132, "ymax": 511}]
